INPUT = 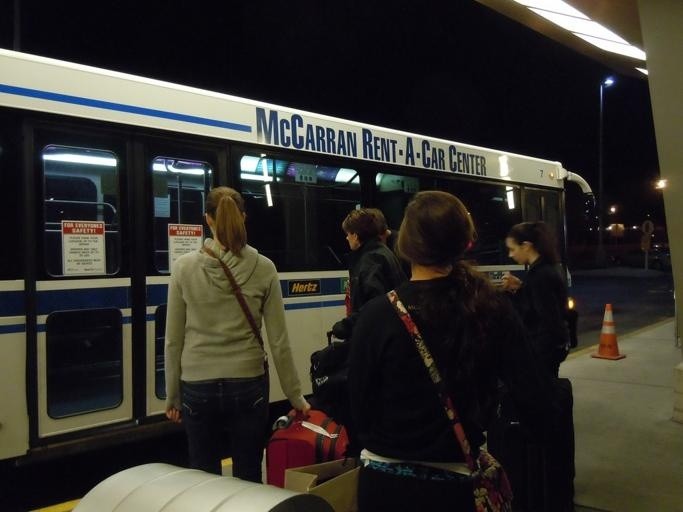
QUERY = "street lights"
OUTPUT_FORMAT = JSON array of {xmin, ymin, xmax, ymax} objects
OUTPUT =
[
  {"xmin": 597, "ymin": 75, "xmax": 618, "ymax": 253},
  {"xmin": 609, "ymin": 205, "xmax": 618, "ymax": 250}
]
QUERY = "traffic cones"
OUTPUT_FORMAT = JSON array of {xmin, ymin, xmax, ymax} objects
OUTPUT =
[{"xmin": 589, "ymin": 301, "xmax": 629, "ymax": 361}]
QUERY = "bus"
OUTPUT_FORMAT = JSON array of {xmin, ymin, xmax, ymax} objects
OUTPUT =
[{"xmin": 0, "ymin": 51, "xmax": 608, "ymax": 483}]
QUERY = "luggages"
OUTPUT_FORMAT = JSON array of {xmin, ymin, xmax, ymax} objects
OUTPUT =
[
  {"xmin": 309, "ymin": 329, "xmax": 350, "ymax": 420},
  {"xmin": 485, "ymin": 376, "xmax": 575, "ymax": 506},
  {"xmin": 265, "ymin": 407, "xmax": 348, "ymax": 489}
]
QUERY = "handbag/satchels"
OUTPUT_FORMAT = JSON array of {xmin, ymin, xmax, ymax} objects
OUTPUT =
[
  {"xmin": 568, "ymin": 308, "xmax": 577, "ymax": 348},
  {"xmin": 470, "ymin": 447, "xmax": 513, "ymax": 512}
]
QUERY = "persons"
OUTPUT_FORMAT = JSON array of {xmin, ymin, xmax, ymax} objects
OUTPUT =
[
  {"xmin": 347, "ymin": 190, "xmax": 562, "ymax": 511},
  {"xmin": 164, "ymin": 186, "xmax": 312, "ymax": 484},
  {"xmin": 332, "ymin": 206, "xmax": 405, "ymax": 340},
  {"xmin": 499, "ymin": 218, "xmax": 569, "ymax": 378}
]
